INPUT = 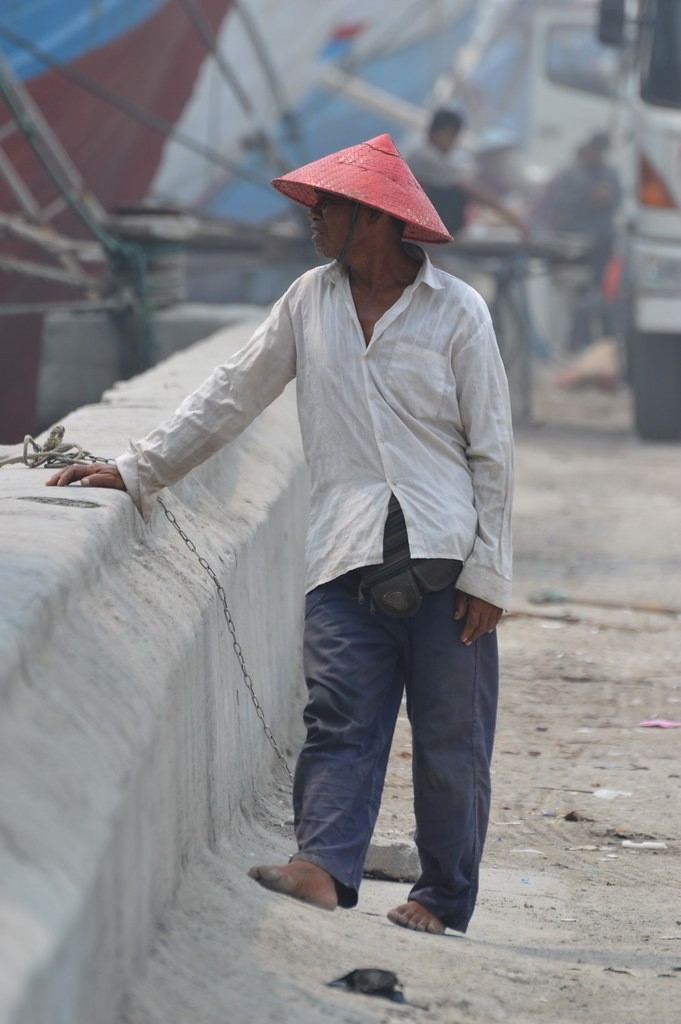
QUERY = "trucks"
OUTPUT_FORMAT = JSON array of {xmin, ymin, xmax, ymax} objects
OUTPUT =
[{"xmin": 527, "ymin": 0, "xmax": 681, "ymax": 442}]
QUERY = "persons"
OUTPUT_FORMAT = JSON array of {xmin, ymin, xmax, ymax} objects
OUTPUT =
[
  {"xmin": 45, "ymin": 134, "xmax": 515, "ymax": 935},
  {"xmin": 400, "ymin": 109, "xmax": 622, "ymax": 291}
]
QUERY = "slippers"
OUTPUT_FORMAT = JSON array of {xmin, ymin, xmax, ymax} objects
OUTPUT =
[{"xmin": 335, "ymin": 964, "xmax": 403, "ymax": 1009}]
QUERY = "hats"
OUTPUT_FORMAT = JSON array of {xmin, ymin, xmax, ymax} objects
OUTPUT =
[{"xmin": 269, "ymin": 135, "xmax": 453, "ymax": 244}]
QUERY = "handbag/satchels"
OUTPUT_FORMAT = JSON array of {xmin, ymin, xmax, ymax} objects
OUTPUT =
[{"xmin": 354, "ymin": 505, "xmax": 464, "ymax": 621}]
